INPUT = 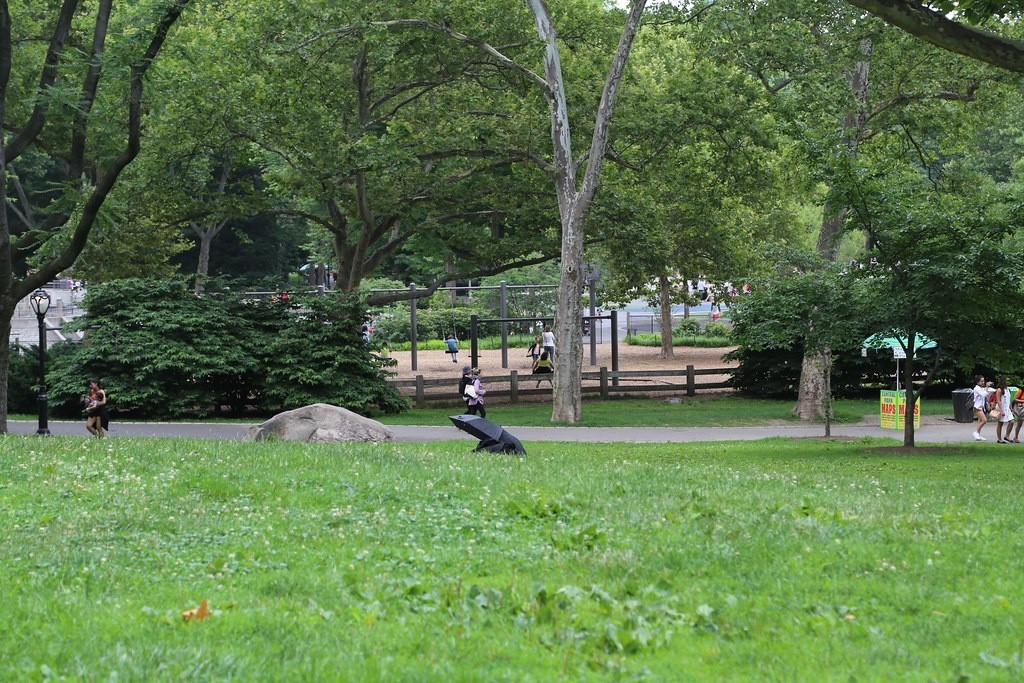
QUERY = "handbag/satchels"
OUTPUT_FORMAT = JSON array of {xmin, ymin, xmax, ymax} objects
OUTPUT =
[
  {"xmin": 1011, "ymin": 402, "xmax": 1024, "ymax": 418},
  {"xmin": 464, "ymin": 380, "xmax": 479, "ymax": 400},
  {"xmin": 990, "ymin": 410, "xmax": 1001, "ymax": 419}
]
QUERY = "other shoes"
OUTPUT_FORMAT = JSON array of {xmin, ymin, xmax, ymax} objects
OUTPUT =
[
  {"xmin": 99, "ymin": 430, "xmax": 104, "ymax": 441},
  {"xmin": 1004, "ymin": 437, "xmax": 1014, "ymax": 442},
  {"xmin": 95, "ymin": 430, "xmax": 99, "ymax": 440},
  {"xmin": 997, "ymin": 440, "xmax": 1006, "ymax": 444},
  {"xmin": 1014, "ymin": 438, "xmax": 1020, "ymax": 443}
]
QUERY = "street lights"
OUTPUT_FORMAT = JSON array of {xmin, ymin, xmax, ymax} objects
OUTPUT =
[{"xmin": 29, "ymin": 288, "xmax": 52, "ymax": 437}]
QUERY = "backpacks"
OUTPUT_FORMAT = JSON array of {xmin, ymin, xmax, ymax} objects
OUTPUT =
[
  {"xmin": 986, "ymin": 388, "xmax": 1004, "ymax": 412},
  {"xmin": 459, "ymin": 378, "xmax": 471, "ymax": 394},
  {"xmin": 965, "ymin": 390, "xmax": 974, "ymax": 414}
]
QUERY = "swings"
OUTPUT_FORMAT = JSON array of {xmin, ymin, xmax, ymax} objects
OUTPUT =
[
  {"xmin": 525, "ymin": 287, "xmax": 542, "ymax": 358},
  {"xmin": 465, "ymin": 289, "xmax": 482, "ymax": 358},
  {"xmin": 436, "ymin": 290, "xmax": 458, "ymax": 354},
  {"xmin": 377, "ymin": 301, "xmax": 392, "ymax": 361}
]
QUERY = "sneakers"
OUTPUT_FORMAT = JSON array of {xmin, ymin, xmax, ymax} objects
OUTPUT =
[
  {"xmin": 975, "ymin": 437, "xmax": 986, "ymax": 441},
  {"xmin": 973, "ymin": 432, "xmax": 980, "ymax": 440}
]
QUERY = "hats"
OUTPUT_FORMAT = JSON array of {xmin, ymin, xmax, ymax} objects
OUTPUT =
[{"xmin": 462, "ymin": 365, "xmax": 472, "ymax": 374}]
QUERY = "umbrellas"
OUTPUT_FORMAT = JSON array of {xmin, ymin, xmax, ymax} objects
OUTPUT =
[
  {"xmin": 300, "ymin": 262, "xmax": 334, "ymax": 271},
  {"xmin": 860, "ymin": 328, "xmax": 937, "ymax": 391}
]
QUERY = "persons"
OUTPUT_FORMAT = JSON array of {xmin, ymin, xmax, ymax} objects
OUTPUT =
[
  {"xmin": 80, "ymin": 379, "xmax": 108, "ymax": 438},
  {"xmin": 444, "ymin": 335, "xmax": 487, "ymax": 418},
  {"xmin": 362, "ymin": 325, "xmax": 393, "ymax": 360},
  {"xmin": 67, "ymin": 276, "xmax": 84, "ymax": 293},
  {"xmin": 973, "ymin": 375, "xmax": 1024, "ymax": 444},
  {"xmin": 695, "ymin": 278, "xmax": 753, "ymax": 322},
  {"xmin": 268, "ymin": 288, "xmax": 291, "ymax": 304},
  {"xmin": 524, "ymin": 325, "xmax": 556, "ymax": 388}
]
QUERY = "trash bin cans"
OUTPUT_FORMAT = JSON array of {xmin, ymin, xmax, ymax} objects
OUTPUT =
[
  {"xmin": 952, "ymin": 390, "xmax": 974, "ymax": 422},
  {"xmin": 985, "ymin": 389, "xmax": 997, "ymax": 421},
  {"xmin": 1007, "ymin": 387, "xmax": 1020, "ymax": 421}
]
